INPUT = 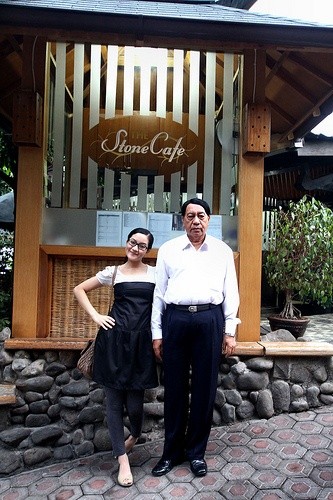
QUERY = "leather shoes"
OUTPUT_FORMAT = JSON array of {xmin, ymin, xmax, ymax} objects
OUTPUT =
[
  {"xmin": 151, "ymin": 455, "xmax": 186, "ymax": 477},
  {"xmin": 187, "ymin": 456, "xmax": 206, "ymax": 477}
]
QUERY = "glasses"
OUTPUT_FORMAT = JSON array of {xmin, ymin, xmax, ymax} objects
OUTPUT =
[{"xmin": 128, "ymin": 238, "xmax": 148, "ymax": 251}]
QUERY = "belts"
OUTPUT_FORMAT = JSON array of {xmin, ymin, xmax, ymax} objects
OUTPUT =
[{"xmin": 167, "ymin": 303, "xmax": 216, "ymax": 312}]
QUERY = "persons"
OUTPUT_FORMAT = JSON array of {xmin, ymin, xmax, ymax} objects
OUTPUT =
[
  {"xmin": 151, "ymin": 197, "xmax": 240, "ymax": 478},
  {"xmin": 73, "ymin": 228, "xmax": 157, "ymax": 486}
]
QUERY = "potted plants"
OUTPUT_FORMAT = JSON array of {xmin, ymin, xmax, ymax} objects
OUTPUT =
[{"xmin": 262, "ymin": 194, "xmax": 333, "ymax": 339}]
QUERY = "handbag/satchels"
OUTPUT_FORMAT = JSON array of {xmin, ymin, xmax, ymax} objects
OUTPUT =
[{"xmin": 77, "ymin": 339, "xmax": 96, "ymax": 380}]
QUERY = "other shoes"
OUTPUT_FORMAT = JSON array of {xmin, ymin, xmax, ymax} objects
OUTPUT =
[
  {"xmin": 118, "ymin": 453, "xmax": 135, "ymax": 487},
  {"xmin": 123, "ymin": 434, "xmax": 139, "ymax": 453}
]
